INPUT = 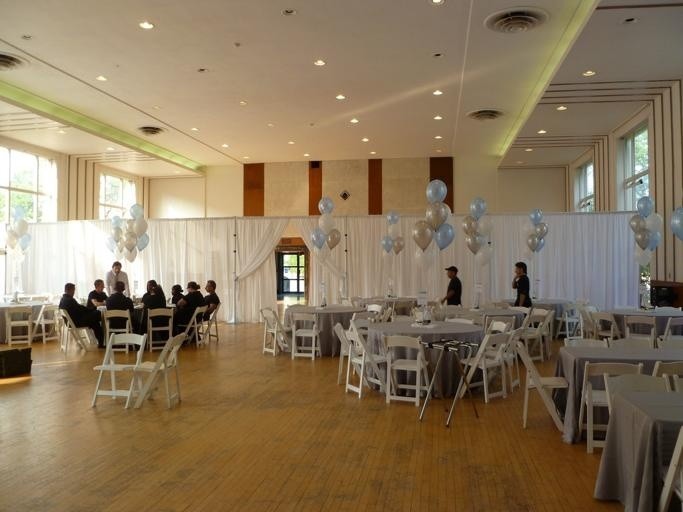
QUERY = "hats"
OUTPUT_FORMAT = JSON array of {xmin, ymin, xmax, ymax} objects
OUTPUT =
[{"xmin": 445, "ymin": 266, "xmax": 458, "ymax": 272}]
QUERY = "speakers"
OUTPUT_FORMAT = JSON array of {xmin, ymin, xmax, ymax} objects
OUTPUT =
[{"xmin": 650, "ymin": 285, "xmax": 674, "ymax": 307}]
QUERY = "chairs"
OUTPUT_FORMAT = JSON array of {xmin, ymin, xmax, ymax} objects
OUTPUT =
[
  {"xmin": 124, "ymin": 331, "xmax": 186, "ymax": 411},
  {"xmin": 552, "ymin": 306, "xmax": 682, "ymax": 510},
  {"xmin": 89, "ymin": 332, "xmax": 147, "ymax": 411},
  {"xmin": 0, "ymin": 295, "xmax": 219, "ymax": 355},
  {"xmin": 260, "ymin": 294, "xmax": 573, "ymax": 427}
]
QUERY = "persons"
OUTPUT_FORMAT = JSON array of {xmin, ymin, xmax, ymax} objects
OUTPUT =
[
  {"xmin": 103, "ymin": 261, "xmax": 131, "ymax": 298},
  {"xmin": 172, "ymin": 281, "xmax": 204, "ymax": 337},
  {"xmin": 139, "ymin": 284, "xmax": 166, "ymax": 333},
  {"xmin": 171, "ymin": 284, "xmax": 184, "ymax": 304},
  {"xmin": 510, "ymin": 262, "xmax": 532, "ymax": 308},
  {"xmin": 139, "ymin": 280, "xmax": 157, "ymax": 306},
  {"xmin": 105, "ymin": 281, "xmax": 134, "ymax": 329},
  {"xmin": 85, "ymin": 279, "xmax": 108, "ymax": 321},
  {"xmin": 57, "ymin": 283, "xmax": 105, "ymax": 349},
  {"xmin": 440, "ymin": 265, "xmax": 462, "ymax": 305},
  {"xmin": 192, "ymin": 280, "xmax": 219, "ymax": 342}
]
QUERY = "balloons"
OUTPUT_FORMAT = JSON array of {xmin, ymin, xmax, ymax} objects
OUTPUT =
[
  {"xmin": 526, "ymin": 208, "xmax": 547, "ymax": 253},
  {"xmin": 104, "ymin": 204, "xmax": 148, "ymax": 262},
  {"xmin": 311, "ymin": 197, "xmax": 340, "ymax": 250},
  {"xmin": 627, "ymin": 195, "xmax": 682, "ymax": 268},
  {"xmin": 380, "ymin": 179, "xmax": 486, "ymax": 255},
  {"xmin": 5, "ymin": 205, "xmax": 31, "ymax": 253}
]
